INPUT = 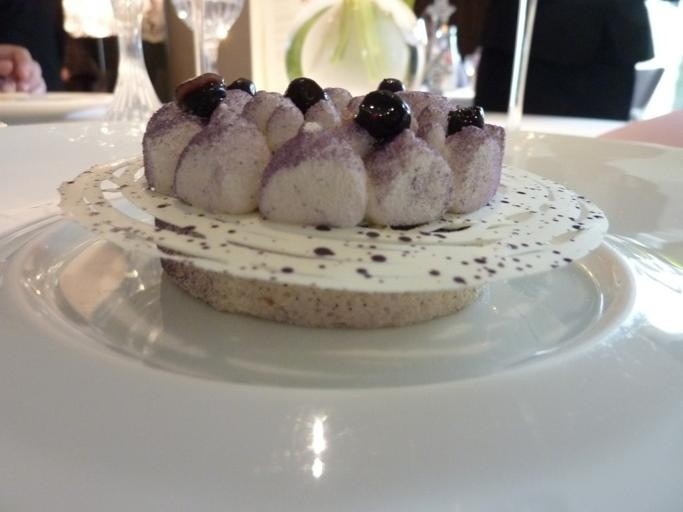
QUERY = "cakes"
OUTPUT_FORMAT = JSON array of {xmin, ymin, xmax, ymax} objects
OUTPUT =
[{"xmin": 56, "ymin": 71, "xmax": 609, "ymax": 329}]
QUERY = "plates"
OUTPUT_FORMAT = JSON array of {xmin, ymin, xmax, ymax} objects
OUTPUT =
[{"xmin": 0, "ymin": 119, "xmax": 683, "ymax": 511}]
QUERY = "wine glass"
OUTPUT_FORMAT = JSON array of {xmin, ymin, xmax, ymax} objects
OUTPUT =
[{"xmin": 169, "ymin": 0, "xmax": 246, "ymax": 78}]
